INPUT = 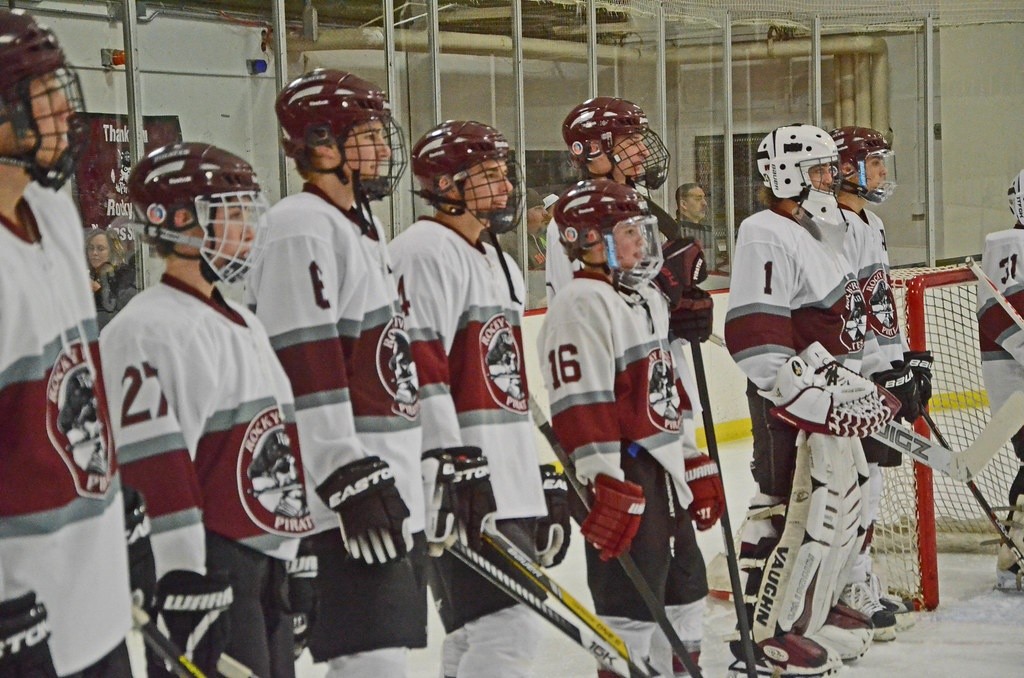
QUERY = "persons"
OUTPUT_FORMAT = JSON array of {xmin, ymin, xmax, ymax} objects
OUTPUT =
[
  {"xmin": 0, "ymin": 0, "xmax": 159, "ymax": 678},
  {"xmin": 248, "ymin": 68, "xmax": 431, "ymax": 678},
  {"xmin": 724, "ymin": 123, "xmax": 934, "ymax": 678},
  {"xmin": 83, "ymin": 227, "xmax": 138, "ymax": 338},
  {"xmin": 674, "ymin": 184, "xmax": 717, "ymax": 274},
  {"xmin": 536, "ymin": 96, "xmax": 715, "ymax": 678},
  {"xmin": 480, "ymin": 187, "xmax": 559, "ymax": 271},
  {"xmin": 96, "ymin": 141, "xmax": 315, "ymax": 678},
  {"xmin": 384, "ymin": 121, "xmax": 572, "ymax": 678},
  {"xmin": 975, "ymin": 167, "xmax": 1024, "ymax": 593}
]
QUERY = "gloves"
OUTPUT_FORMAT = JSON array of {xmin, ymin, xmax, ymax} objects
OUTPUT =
[
  {"xmin": 287, "ymin": 572, "xmax": 318, "ymax": 661},
  {"xmin": 155, "ymin": 569, "xmax": 231, "ymax": 678},
  {"xmin": 871, "ymin": 361, "xmax": 922, "ymax": 425},
  {"xmin": 669, "ymin": 287, "xmax": 714, "ymax": 343},
  {"xmin": 0, "ymin": 603, "xmax": 59, "ymax": 678},
  {"xmin": 655, "ymin": 234, "xmax": 709, "ymax": 300},
  {"xmin": 428, "ymin": 446, "xmax": 498, "ymax": 554},
  {"xmin": 902, "ymin": 351, "xmax": 934, "ymax": 406},
  {"xmin": 581, "ymin": 472, "xmax": 645, "ymax": 560},
  {"xmin": 532, "ymin": 463, "xmax": 571, "ymax": 568},
  {"xmin": 683, "ymin": 453, "xmax": 725, "ymax": 530},
  {"xmin": 321, "ymin": 459, "xmax": 414, "ymax": 567}
]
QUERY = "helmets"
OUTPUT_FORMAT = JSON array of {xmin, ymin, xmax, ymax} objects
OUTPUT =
[
  {"xmin": 829, "ymin": 125, "xmax": 897, "ymax": 205},
  {"xmin": 554, "ymin": 179, "xmax": 664, "ymax": 290},
  {"xmin": 275, "ymin": 66, "xmax": 409, "ymax": 202},
  {"xmin": 561, "ymin": 95, "xmax": 670, "ymax": 189},
  {"xmin": 1007, "ymin": 167, "xmax": 1024, "ymax": 226},
  {"xmin": 410, "ymin": 119, "xmax": 524, "ymax": 233},
  {"xmin": 0, "ymin": 9, "xmax": 91, "ymax": 190},
  {"xmin": 127, "ymin": 142, "xmax": 266, "ymax": 285},
  {"xmin": 757, "ymin": 124, "xmax": 840, "ymax": 226}
]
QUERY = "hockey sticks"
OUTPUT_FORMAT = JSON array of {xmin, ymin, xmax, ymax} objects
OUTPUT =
[
  {"xmin": 919, "ymin": 402, "xmax": 1024, "ymax": 571},
  {"xmin": 964, "ymin": 255, "xmax": 1024, "ymax": 332},
  {"xmin": 133, "ymin": 604, "xmax": 207, "ymax": 678},
  {"xmin": 708, "ymin": 334, "xmax": 1024, "ymax": 485},
  {"xmin": 443, "ymin": 536, "xmax": 651, "ymax": 678},
  {"xmin": 525, "ymin": 390, "xmax": 704, "ymax": 678},
  {"xmin": 687, "ymin": 338, "xmax": 759, "ymax": 678},
  {"xmin": 480, "ymin": 521, "xmax": 663, "ymax": 678}
]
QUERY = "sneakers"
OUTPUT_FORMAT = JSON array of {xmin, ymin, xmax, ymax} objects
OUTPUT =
[
  {"xmin": 728, "ymin": 616, "xmax": 828, "ymax": 677},
  {"xmin": 838, "ymin": 578, "xmax": 897, "ymax": 640},
  {"xmin": 861, "ymin": 569, "xmax": 918, "ymax": 631}
]
